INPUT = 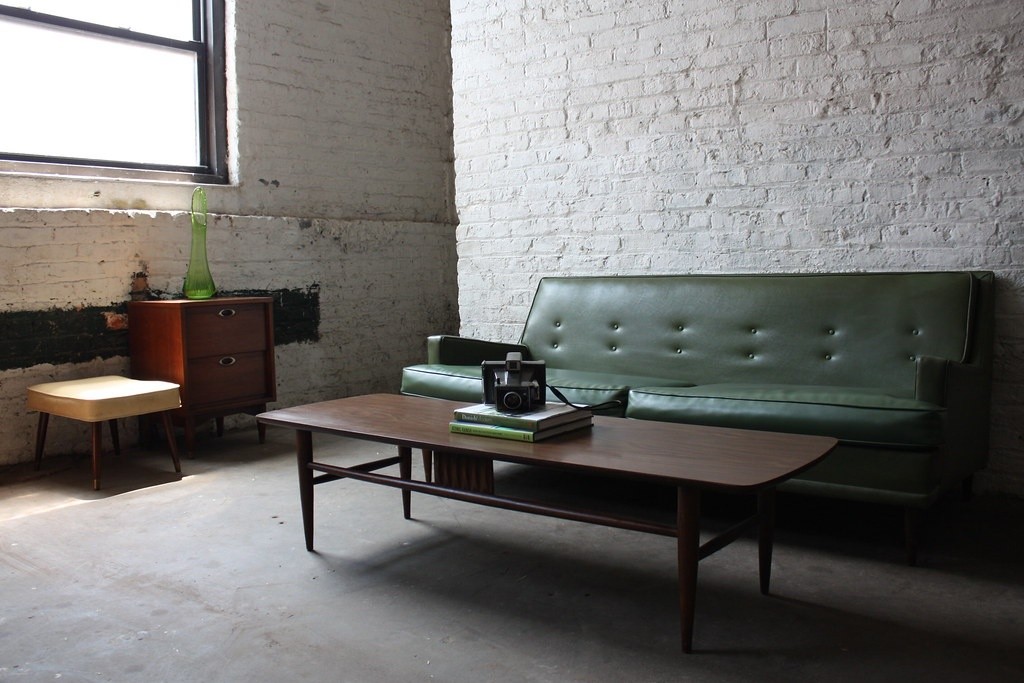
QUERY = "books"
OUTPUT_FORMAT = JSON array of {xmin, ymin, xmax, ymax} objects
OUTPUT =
[{"xmin": 449, "ymin": 400, "xmax": 594, "ymax": 443}]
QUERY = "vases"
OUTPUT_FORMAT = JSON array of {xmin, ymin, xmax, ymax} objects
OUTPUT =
[{"xmin": 181, "ymin": 186, "xmax": 216, "ymax": 300}]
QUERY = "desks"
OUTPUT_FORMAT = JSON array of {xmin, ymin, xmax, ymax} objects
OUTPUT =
[{"xmin": 257, "ymin": 394, "xmax": 840, "ymax": 655}]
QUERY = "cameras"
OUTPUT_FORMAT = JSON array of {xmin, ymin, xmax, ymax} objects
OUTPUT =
[{"xmin": 481, "ymin": 352, "xmax": 546, "ymax": 413}]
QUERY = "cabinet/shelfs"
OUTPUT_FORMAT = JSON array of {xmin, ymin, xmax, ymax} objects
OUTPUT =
[{"xmin": 129, "ymin": 295, "xmax": 277, "ymax": 456}]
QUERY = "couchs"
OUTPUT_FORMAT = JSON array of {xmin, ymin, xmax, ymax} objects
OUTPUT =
[{"xmin": 400, "ymin": 270, "xmax": 997, "ymax": 566}]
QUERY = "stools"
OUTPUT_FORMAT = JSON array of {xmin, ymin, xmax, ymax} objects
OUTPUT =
[{"xmin": 28, "ymin": 374, "xmax": 181, "ymax": 492}]
